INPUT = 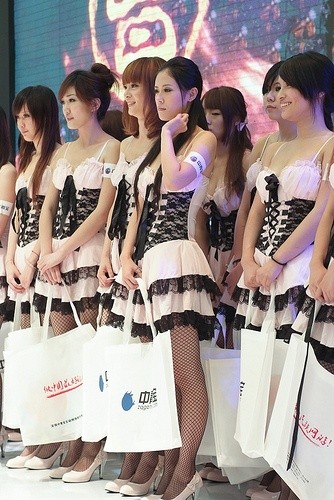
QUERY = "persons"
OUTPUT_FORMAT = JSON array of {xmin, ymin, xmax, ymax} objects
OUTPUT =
[{"xmin": 0, "ymin": 50, "xmax": 334, "ymax": 500}]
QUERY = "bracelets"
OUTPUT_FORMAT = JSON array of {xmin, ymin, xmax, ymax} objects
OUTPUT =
[
  {"xmin": 271, "ymin": 247, "xmax": 287, "ymax": 266},
  {"xmin": 232, "ymin": 258, "xmax": 241, "ymax": 264}
]
